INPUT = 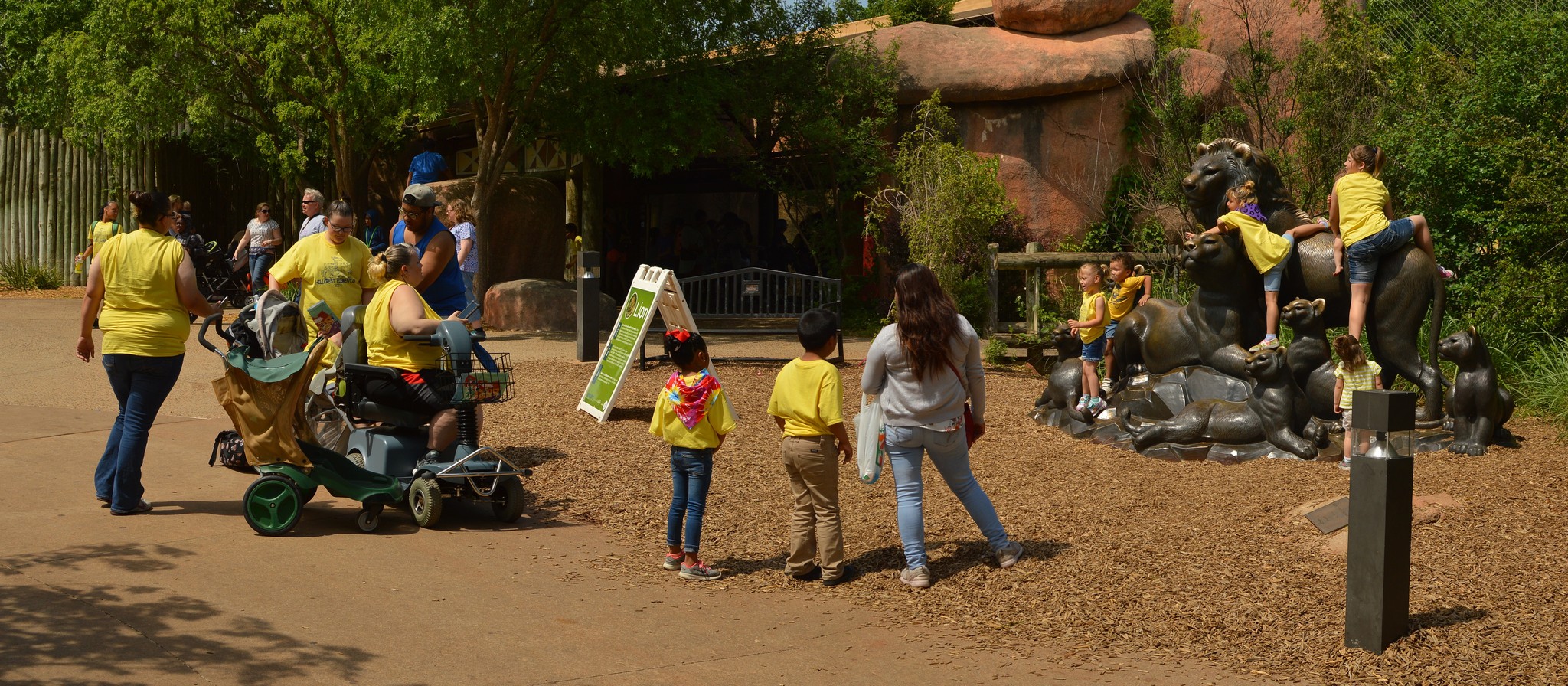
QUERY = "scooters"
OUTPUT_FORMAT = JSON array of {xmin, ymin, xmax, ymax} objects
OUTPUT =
[{"xmin": 332, "ymin": 302, "xmax": 533, "ymax": 529}]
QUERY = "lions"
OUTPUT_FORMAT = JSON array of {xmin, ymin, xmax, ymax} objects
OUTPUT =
[{"xmin": 1031, "ymin": 135, "xmax": 1524, "ymax": 462}]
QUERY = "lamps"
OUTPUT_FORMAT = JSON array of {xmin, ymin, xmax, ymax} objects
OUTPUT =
[{"xmin": 1351, "ymin": 428, "xmax": 1414, "ymax": 459}]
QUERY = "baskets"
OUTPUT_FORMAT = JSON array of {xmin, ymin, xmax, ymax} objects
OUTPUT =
[{"xmin": 435, "ymin": 352, "xmax": 516, "ymax": 404}]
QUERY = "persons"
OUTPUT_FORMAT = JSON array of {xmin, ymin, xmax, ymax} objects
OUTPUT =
[
  {"xmin": 766, "ymin": 308, "xmax": 855, "ymax": 586},
  {"xmin": 361, "ymin": 244, "xmax": 483, "ymax": 475},
  {"xmin": 74, "ymin": 195, "xmax": 207, "ymax": 329},
  {"xmin": 231, "ymin": 183, "xmax": 487, "ymax": 340},
  {"xmin": 406, "ymin": 138, "xmax": 453, "ymax": 188},
  {"xmin": 1327, "ymin": 145, "xmax": 1454, "ymax": 342},
  {"xmin": 1102, "ymin": 252, "xmax": 1151, "ymax": 394},
  {"xmin": 1185, "ymin": 180, "xmax": 1331, "ymax": 351},
  {"xmin": 649, "ymin": 328, "xmax": 736, "ymax": 580},
  {"xmin": 564, "ymin": 223, "xmax": 582, "ymax": 269},
  {"xmin": 862, "ymin": 264, "xmax": 1024, "ymax": 588},
  {"xmin": 1067, "ymin": 262, "xmax": 1111, "ymax": 416},
  {"xmin": 268, "ymin": 199, "xmax": 386, "ymax": 428},
  {"xmin": 76, "ymin": 189, "xmax": 224, "ymax": 516},
  {"xmin": 1333, "ymin": 334, "xmax": 1383, "ymax": 471}
]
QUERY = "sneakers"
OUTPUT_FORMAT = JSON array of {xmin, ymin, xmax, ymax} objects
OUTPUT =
[
  {"xmin": 1076, "ymin": 396, "xmax": 1107, "ymax": 417},
  {"xmin": 663, "ymin": 547, "xmax": 686, "ymax": 570},
  {"xmin": 1249, "ymin": 338, "xmax": 1280, "ymax": 352},
  {"xmin": 900, "ymin": 565, "xmax": 930, "ymax": 587},
  {"xmin": 1102, "ymin": 376, "xmax": 1115, "ymax": 394},
  {"xmin": 1338, "ymin": 459, "xmax": 1350, "ymax": 471},
  {"xmin": 679, "ymin": 558, "xmax": 721, "ymax": 580},
  {"xmin": 470, "ymin": 330, "xmax": 487, "ymax": 340},
  {"xmin": 1312, "ymin": 217, "xmax": 1331, "ymax": 231},
  {"xmin": 995, "ymin": 541, "xmax": 1023, "ymax": 568},
  {"xmin": 1438, "ymin": 263, "xmax": 1454, "ymax": 279}
]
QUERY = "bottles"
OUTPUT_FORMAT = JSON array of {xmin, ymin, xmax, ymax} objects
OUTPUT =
[
  {"xmin": 74, "ymin": 253, "xmax": 84, "ymax": 274},
  {"xmin": 254, "ymin": 295, "xmax": 260, "ymax": 312}
]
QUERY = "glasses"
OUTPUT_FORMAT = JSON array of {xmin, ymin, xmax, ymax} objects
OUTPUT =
[
  {"xmin": 446, "ymin": 209, "xmax": 454, "ymax": 212},
  {"xmin": 398, "ymin": 206, "xmax": 422, "ymax": 220},
  {"xmin": 159, "ymin": 210, "xmax": 177, "ymax": 221},
  {"xmin": 302, "ymin": 200, "xmax": 315, "ymax": 204},
  {"xmin": 260, "ymin": 209, "xmax": 271, "ymax": 214},
  {"xmin": 328, "ymin": 217, "xmax": 353, "ymax": 232}
]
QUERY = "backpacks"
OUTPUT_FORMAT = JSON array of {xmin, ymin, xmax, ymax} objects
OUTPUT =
[
  {"xmin": 174, "ymin": 233, "xmax": 208, "ymax": 275},
  {"xmin": 220, "ymin": 430, "xmax": 247, "ymax": 466}
]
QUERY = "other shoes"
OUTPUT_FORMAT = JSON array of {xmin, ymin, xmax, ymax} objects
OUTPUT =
[
  {"xmin": 93, "ymin": 318, "xmax": 99, "ymax": 329},
  {"xmin": 412, "ymin": 450, "xmax": 442, "ymax": 476},
  {"xmin": 97, "ymin": 496, "xmax": 151, "ymax": 515},
  {"xmin": 784, "ymin": 566, "xmax": 821, "ymax": 578},
  {"xmin": 823, "ymin": 565, "xmax": 856, "ymax": 586}
]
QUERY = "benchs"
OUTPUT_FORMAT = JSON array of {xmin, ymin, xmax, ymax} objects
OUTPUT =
[{"xmin": 640, "ymin": 267, "xmax": 844, "ymax": 371}]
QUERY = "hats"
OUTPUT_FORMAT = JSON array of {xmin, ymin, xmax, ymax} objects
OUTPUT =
[{"xmin": 402, "ymin": 183, "xmax": 442, "ymax": 207}]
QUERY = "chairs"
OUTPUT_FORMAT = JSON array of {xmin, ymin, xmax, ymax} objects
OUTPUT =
[{"xmin": 342, "ymin": 304, "xmax": 433, "ymax": 427}]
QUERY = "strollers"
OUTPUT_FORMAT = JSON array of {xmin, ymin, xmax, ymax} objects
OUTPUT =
[
  {"xmin": 198, "ymin": 288, "xmax": 355, "ymax": 451},
  {"xmin": 239, "ymin": 304, "xmax": 407, "ymax": 537},
  {"xmin": 188, "ymin": 230, "xmax": 255, "ymax": 324}
]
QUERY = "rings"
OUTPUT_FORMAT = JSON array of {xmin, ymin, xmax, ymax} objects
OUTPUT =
[
  {"xmin": 79, "ymin": 352, "xmax": 83, "ymax": 355},
  {"xmin": 76, "ymin": 351, "xmax": 79, "ymax": 353}
]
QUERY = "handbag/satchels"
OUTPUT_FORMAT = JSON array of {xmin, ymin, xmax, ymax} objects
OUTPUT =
[
  {"xmin": 964, "ymin": 402, "xmax": 974, "ymax": 451},
  {"xmin": 853, "ymin": 392, "xmax": 885, "ymax": 484}
]
